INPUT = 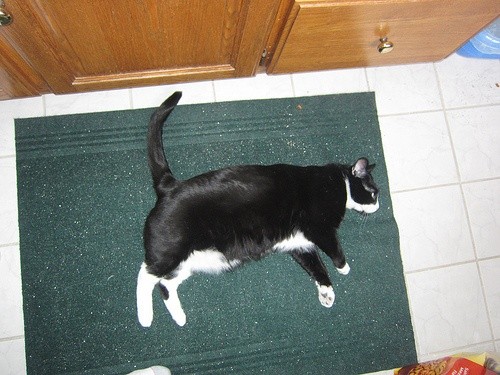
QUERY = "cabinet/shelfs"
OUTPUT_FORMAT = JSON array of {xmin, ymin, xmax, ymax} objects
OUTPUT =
[{"xmin": 258, "ymin": 0, "xmax": 500, "ymax": 101}]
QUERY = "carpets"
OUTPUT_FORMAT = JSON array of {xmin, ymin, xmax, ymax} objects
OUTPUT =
[{"xmin": 14, "ymin": 92, "xmax": 419, "ymax": 375}]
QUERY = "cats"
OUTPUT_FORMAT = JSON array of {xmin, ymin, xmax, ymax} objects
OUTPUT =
[{"xmin": 136, "ymin": 92, "xmax": 380, "ymax": 328}]
